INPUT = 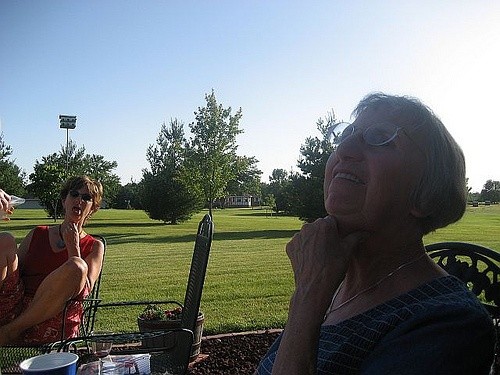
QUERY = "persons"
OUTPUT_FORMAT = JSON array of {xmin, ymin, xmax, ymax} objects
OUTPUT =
[
  {"xmin": 253, "ymin": 92, "xmax": 495, "ymax": 374},
  {"xmin": 0, "ymin": 170, "xmax": 104, "ymax": 345}
]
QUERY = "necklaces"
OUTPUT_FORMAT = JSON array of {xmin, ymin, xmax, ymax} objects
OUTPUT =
[
  {"xmin": 321, "ymin": 252, "xmax": 427, "ymax": 324},
  {"xmin": 58, "ymin": 224, "xmax": 83, "ymax": 248}
]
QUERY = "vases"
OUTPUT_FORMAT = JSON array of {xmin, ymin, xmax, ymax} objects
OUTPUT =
[{"xmin": 137, "ymin": 313, "xmax": 204, "ymax": 361}]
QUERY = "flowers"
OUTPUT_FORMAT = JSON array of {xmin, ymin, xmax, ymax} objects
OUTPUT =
[{"xmin": 137, "ymin": 305, "xmax": 207, "ymax": 320}]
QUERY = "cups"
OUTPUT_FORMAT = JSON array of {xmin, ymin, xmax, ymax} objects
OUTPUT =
[{"xmin": 18, "ymin": 352, "xmax": 79, "ymax": 375}]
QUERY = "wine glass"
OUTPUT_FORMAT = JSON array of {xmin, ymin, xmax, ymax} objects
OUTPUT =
[{"xmin": 90, "ymin": 330, "xmax": 114, "ymax": 375}]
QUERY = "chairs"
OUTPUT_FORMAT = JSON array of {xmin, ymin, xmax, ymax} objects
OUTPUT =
[
  {"xmin": 0, "ymin": 214, "xmax": 214, "ymax": 375},
  {"xmin": 423, "ymin": 242, "xmax": 500, "ymax": 375}
]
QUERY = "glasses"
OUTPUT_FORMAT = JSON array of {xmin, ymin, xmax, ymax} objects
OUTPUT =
[
  {"xmin": 69, "ymin": 191, "xmax": 92, "ymax": 201},
  {"xmin": 325, "ymin": 121, "xmax": 427, "ymax": 156}
]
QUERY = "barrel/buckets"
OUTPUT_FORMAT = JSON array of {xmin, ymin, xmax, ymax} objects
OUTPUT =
[{"xmin": 136, "ymin": 310, "xmax": 204, "ymax": 364}]
